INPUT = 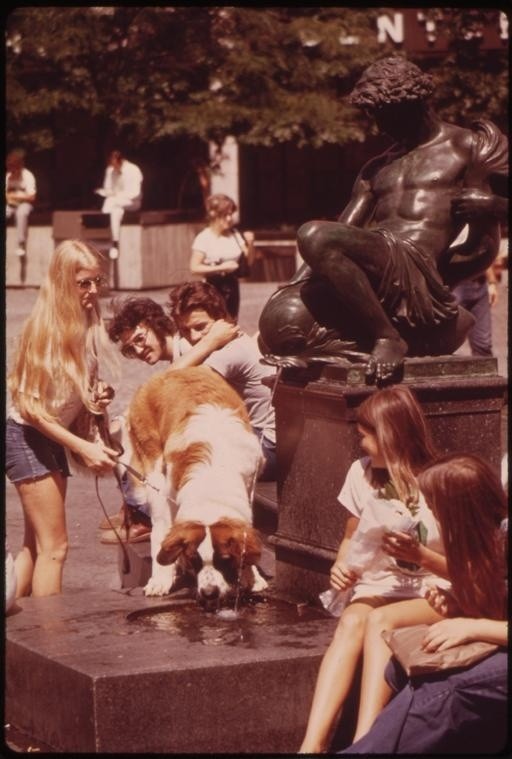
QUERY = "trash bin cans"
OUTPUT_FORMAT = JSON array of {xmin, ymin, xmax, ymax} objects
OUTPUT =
[{"xmin": 52, "ymin": 211, "xmax": 112, "ymax": 294}]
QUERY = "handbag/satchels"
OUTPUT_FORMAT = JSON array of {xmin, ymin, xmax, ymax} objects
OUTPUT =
[{"xmin": 381, "ymin": 624, "xmax": 499, "ymax": 678}]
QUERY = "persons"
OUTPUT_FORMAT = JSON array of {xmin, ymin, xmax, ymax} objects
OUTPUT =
[
  {"xmin": 255, "ymin": 55, "xmax": 510, "ymax": 377},
  {"xmin": 441, "ymin": 248, "xmax": 501, "ymax": 357},
  {"xmin": 92, "ymin": 151, "xmax": 145, "ymax": 260},
  {"xmin": 201, "ymin": 124, "xmax": 243, "ymax": 228},
  {"xmin": 100, "ymin": 279, "xmax": 277, "ymax": 546},
  {"xmin": 5, "ymin": 149, "xmax": 38, "ymax": 258},
  {"xmin": 104, "ymin": 292, "xmax": 181, "ymax": 370},
  {"xmin": 187, "ymin": 193, "xmax": 261, "ymax": 324},
  {"xmin": 294, "ymin": 385, "xmax": 510, "ymax": 756},
  {"xmin": 6, "ymin": 239, "xmax": 120, "ymax": 595}
]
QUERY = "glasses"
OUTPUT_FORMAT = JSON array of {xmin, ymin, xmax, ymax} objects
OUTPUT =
[
  {"xmin": 122, "ymin": 328, "xmax": 149, "ymax": 359},
  {"xmin": 80, "ymin": 277, "xmax": 104, "ymax": 288}
]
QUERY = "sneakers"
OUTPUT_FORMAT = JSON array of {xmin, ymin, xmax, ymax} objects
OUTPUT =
[
  {"xmin": 16, "ymin": 249, "xmax": 25, "ymax": 257},
  {"xmin": 109, "ymin": 247, "xmax": 119, "ymax": 260}
]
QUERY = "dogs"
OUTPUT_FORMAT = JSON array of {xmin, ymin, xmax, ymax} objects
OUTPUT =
[{"xmin": 115, "ymin": 361, "xmax": 272, "ymax": 603}]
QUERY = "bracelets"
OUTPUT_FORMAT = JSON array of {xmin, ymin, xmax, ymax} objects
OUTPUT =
[{"xmin": 487, "ymin": 281, "xmax": 499, "ymax": 287}]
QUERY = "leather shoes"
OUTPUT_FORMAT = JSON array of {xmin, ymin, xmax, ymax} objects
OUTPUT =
[{"xmin": 100, "ymin": 504, "xmax": 152, "ymax": 543}]
